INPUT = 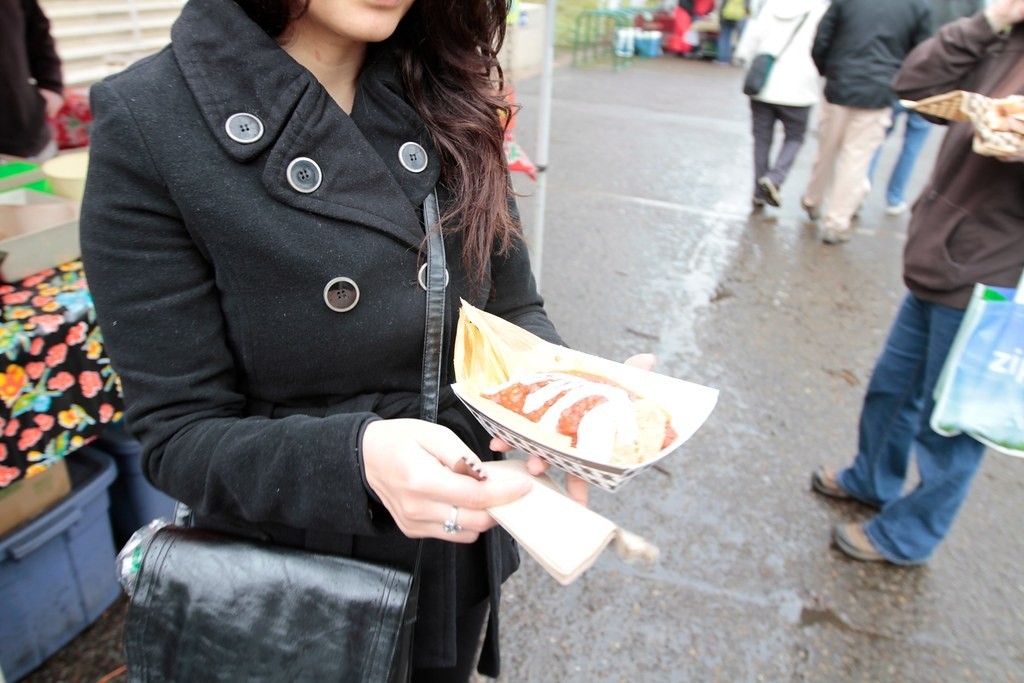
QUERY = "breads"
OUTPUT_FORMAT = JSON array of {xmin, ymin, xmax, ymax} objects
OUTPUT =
[{"xmin": 989, "ymin": 94, "xmax": 1024, "ymax": 136}]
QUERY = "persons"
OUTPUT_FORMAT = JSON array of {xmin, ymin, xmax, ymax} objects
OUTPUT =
[
  {"xmin": 0, "ymin": 0, "xmax": 65, "ymax": 164},
  {"xmin": 677, "ymin": 0, "xmax": 984, "ymax": 244},
  {"xmin": 811, "ymin": 0, "xmax": 1024, "ymax": 565},
  {"xmin": 78, "ymin": 0, "xmax": 656, "ymax": 683}
]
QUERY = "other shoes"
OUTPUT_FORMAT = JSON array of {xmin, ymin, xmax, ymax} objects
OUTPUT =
[
  {"xmin": 757, "ymin": 176, "xmax": 783, "ymax": 207},
  {"xmin": 801, "ymin": 199, "xmax": 818, "ymax": 220},
  {"xmin": 884, "ymin": 202, "xmax": 908, "ymax": 214},
  {"xmin": 811, "ymin": 466, "xmax": 852, "ymax": 500},
  {"xmin": 824, "ymin": 228, "xmax": 851, "ymax": 244},
  {"xmin": 752, "ymin": 196, "xmax": 765, "ymax": 210},
  {"xmin": 833, "ymin": 521, "xmax": 891, "ymax": 562}
]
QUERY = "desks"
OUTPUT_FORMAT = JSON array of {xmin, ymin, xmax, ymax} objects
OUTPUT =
[{"xmin": 0, "ymin": 259, "xmax": 125, "ymax": 489}]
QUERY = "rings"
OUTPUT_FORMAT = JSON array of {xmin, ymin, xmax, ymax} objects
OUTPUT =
[{"xmin": 443, "ymin": 504, "xmax": 463, "ymax": 535}]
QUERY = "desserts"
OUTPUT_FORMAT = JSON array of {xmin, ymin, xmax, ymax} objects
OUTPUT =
[{"xmin": 498, "ymin": 373, "xmax": 678, "ymax": 460}]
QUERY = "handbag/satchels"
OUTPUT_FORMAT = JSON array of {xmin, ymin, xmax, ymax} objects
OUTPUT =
[
  {"xmin": 742, "ymin": 53, "xmax": 775, "ymax": 94},
  {"xmin": 721, "ymin": 0, "xmax": 748, "ymax": 21},
  {"xmin": 928, "ymin": 273, "xmax": 1024, "ymax": 459},
  {"xmin": 123, "ymin": 522, "xmax": 420, "ymax": 683}
]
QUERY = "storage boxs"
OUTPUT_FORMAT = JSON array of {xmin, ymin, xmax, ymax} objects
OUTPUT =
[
  {"xmin": 0, "ymin": 458, "xmax": 71, "ymax": 542},
  {"xmin": 98, "ymin": 430, "xmax": 177, "ymax": 535},
  {"xmin": 0, "ymin": 447, "xmax": 123, "ymax": 682},
  {"xmin": 635, "ymin": 38, "xmax": 660, "ymax": 57}
]
